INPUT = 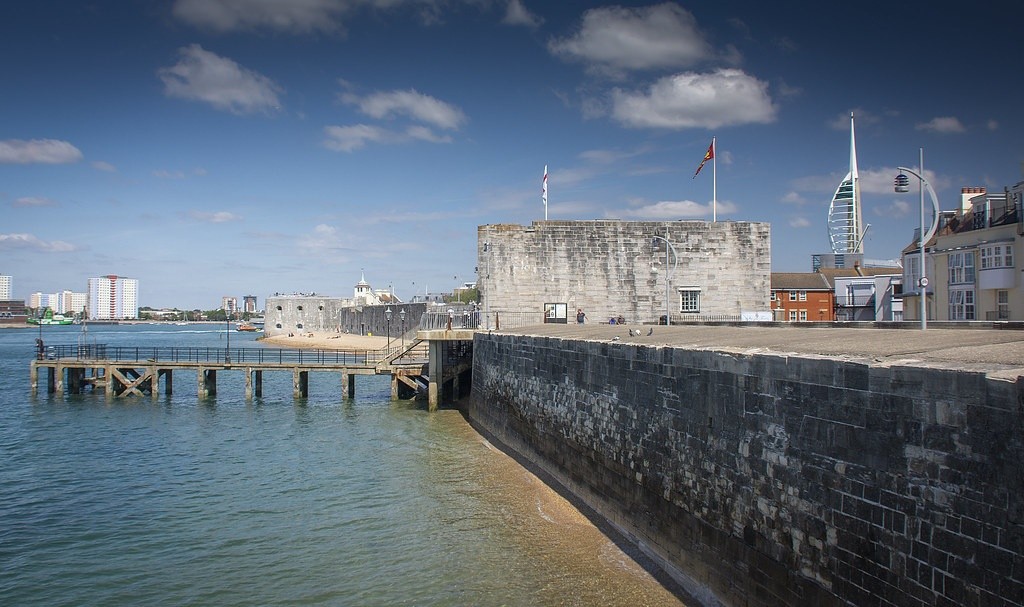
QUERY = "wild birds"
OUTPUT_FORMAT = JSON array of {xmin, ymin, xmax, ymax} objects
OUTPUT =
[{"xmin": 612, "ymin": 327, "xmax": 653, "ymax": 341}]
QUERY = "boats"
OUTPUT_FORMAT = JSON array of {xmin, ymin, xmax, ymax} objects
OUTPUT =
[
  {"xmin": 26, "ymin": 306, "xmax": 75, "ymax": 325},
  {"xmin": 236, "ymin": 321, "xmax": 262, "ymax": 332}
]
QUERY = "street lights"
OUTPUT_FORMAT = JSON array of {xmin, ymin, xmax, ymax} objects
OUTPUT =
[
  {"xmin": 894, "ymin": 148, "xmax": 939, "ymax": 329},
  {"xmin": 385, "ymin": 306, "xmax": 392, "ymax": 365},
  {"xmin": 224, "ymin": 304, "xmax": 232, "ymax": 364},
  {"xmin": 650, "ymin": 226, "xmax": 677, "ymax": 326},
  {"xmin": 400, "ymin": 308, "xmax": 406, "ymax": 358}
]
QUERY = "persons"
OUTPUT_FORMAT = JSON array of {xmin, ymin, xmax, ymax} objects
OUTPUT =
[
  {"xmin": 35, "ymin": 338, "xmax": 44, "ymax": 360},
  {"xmin": 609, "ymin": 318, "xmax": 616, "ymax": 324},
  {"xmin": 447, "ymin": 307, "xmax": 454, "ymax": 330},
  {"xmin": 577, "ymin": 309, "xmax": 589, "ymax": 324}
]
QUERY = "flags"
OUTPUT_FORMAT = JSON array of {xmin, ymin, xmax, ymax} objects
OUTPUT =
[
  {"xmin": 692, "ymin": 140, "xmax": 715, "ymax": 180},
  {"xmin": 542, "ymin": 171, "xmax": 547, "ymax": 205}
]
